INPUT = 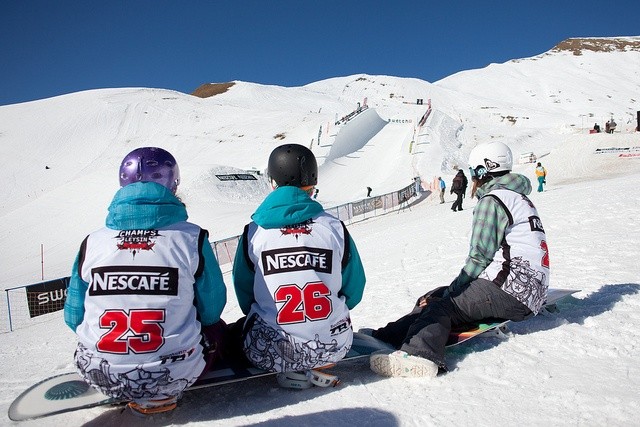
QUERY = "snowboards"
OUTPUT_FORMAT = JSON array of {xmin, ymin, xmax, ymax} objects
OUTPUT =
[
  {"xmin": 8, "ymin": 332, "xmax": 397, "ymax": 421},
  {"xmin": 446, "ymin": 289, "xmax": 582, "ymax": 348}
]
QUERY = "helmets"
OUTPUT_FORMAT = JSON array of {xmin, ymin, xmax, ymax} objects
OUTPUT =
[
  {"xmin": 468, "ymin": 141, "xmax": 515, "ymax": 176},
  {"xmin": 121, "ymin": 146, "xmax": 181, "ymax": 197},
  {"xmin": 267, "ymin": 144, "xmax": 320, "ymax": 191}
]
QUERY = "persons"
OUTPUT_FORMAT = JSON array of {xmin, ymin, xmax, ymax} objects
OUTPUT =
[
  {"xmin": 450, "ymin": 169, "xmax": 468, "ymax": 212},
  {"xmin": 63, "ymin": 148, "xmax": 227, "ymax": 412},
  {"xmin": 437, "ymin": 177, "xmax": 447, "ymax": 204},
  {"xmin": 223, "ymin": 144, "xmax": 367, "ymax": 374},
  {"xmin": 536, "ymin": 163, "xmax": 549, "ymax": 192},
  {"xmin": 359, "ymin": 139, "xmax": 550, "ymax": 383}
]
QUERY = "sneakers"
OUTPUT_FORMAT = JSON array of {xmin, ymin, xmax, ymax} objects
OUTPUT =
[
  {"xmin": 358, "ymin": 327, "xmax": 374, "ymax": 336},
  {"xmin": 370, "ymin": 350, "xmax": 438, "ymax": 379}
]
